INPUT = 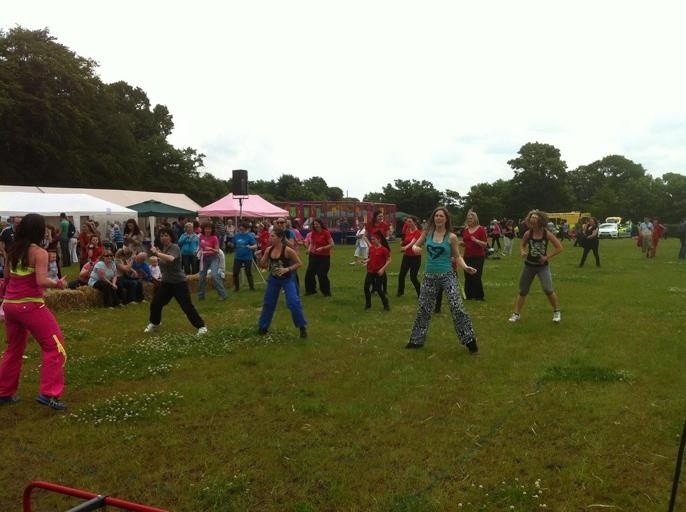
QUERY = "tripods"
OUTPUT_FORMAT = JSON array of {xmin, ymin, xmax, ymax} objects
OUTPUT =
[{"xmin": 236, "ymin": 200, "xmax": 266, "ymax": 284}]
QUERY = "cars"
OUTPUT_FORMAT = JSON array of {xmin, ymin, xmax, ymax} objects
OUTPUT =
[
  {"xmin": 619, "ymin": 224, "xmax": 640, "ymax": 237},
  {"xmin": 597, "ymin": 222, "xmax": 620, "ymax": 239}
]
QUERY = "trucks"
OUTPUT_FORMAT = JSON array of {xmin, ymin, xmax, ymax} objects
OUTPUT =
[{"xmin": 513, "ymin": 210, "xmax": 593, "ymax": 239}]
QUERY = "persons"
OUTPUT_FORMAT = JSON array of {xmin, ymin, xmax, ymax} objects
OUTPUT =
[
  {"xmin": 636, "ymin": 216, "xmax": 666, "ymax": 258},
  {"xmin": 0, "ymin": 206, "xmax": 599, "ymax": 410}
]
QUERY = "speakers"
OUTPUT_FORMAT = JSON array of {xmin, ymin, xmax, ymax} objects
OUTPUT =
[{"xmin": 232, "ymin": 170, "xmax": 248, "ymax": 196}]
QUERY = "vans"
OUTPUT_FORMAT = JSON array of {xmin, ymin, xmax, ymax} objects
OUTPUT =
[{"xmin": 604, "ymin": 216, "xmax": 622, "ymax": 224}]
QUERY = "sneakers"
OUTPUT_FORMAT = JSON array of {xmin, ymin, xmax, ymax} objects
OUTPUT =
[
  {"xmin": 144, "ymin": 322, "xmax": 162, "ymax": 332},
  {"xmin": 36, "ymin": 394, "xmax": 64, "ymax": 409},
  {"xmin": 199, "ymin": 326, "xmax": 208, "ymax": 334},
  {"xmin": 0, "ymin": 396, "xmax": 19, "ymax": 404},
  {"xmin": 509, "ymin": 313, "xmax": 520, "ymax": 322},
  {"xmin": 466, "ymin": 341, "xmax": 479, "ymax": 354},
  {"xmin": 552, "ymin": 311, "xmax": 562, "ymax": 322}
]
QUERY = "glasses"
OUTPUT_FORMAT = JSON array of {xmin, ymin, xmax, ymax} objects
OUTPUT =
[
  {"xmin": 103, "ymin": 254, "xmax": 112, "ymax": 257},
  {"xmin": 277, "ymin": 221, "xmax": 284, "ymax": 224}
]
